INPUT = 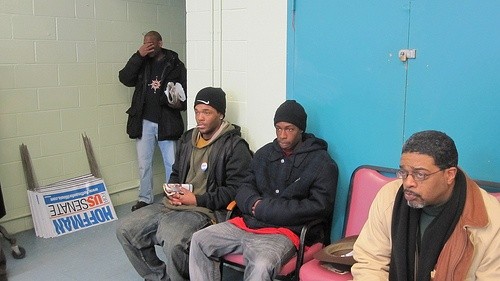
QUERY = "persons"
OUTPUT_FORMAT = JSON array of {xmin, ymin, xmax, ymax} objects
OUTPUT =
[
  {"xmin": 118, "ymin": 31, "xmax": 187, "ymax": 212},
  {"xmin": 350, "ymin": 129, "xmax": 500, "ymax": 281},
  {"xmin": 116, "ymin": 87, "xmax": 252, "ymax": 281},
  {"xmin": 188, "ymin": 100, "xmax": 339, "ymax": 281}
]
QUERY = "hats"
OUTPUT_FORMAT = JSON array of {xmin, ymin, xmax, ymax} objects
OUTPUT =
[
  {"xmin": 274, "ymin": 100, "xmax": 307, "ymax": 131},
  {"xmin": 194, "ymin": 86, "xmax": 227, "ymax": 116},
  {"xmin": 314, "ymin": 234, "xmax": 360, "ymax": 271}
]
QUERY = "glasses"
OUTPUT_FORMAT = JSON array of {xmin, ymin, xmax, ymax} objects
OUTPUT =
[{"xmin": 395, "ymin": 168, "xmax": 444, "ymax": 182}]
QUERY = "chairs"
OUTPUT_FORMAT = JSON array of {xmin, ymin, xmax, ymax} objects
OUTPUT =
[{"xmin": 220, "ymin": 201, "xmax": 330, "ymax": 281}]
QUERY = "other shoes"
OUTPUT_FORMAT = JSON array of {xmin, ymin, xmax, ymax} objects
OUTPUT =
[{"xmin": 131, "ymin": 201, "xmax": 152, "ymax": 212}]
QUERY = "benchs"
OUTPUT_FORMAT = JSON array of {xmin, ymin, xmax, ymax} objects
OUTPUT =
[{"xmin": 299, "ymin": 165, "xmax": 500, "ymax": 281}]
